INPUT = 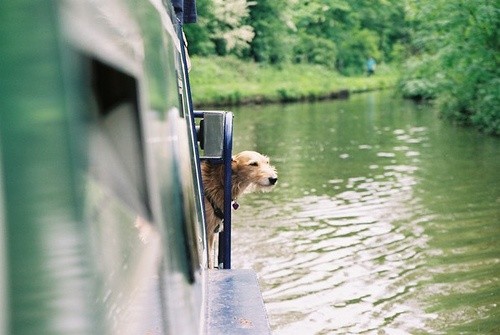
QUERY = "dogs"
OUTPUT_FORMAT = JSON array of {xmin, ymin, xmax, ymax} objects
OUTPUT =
[{"xmin": 200, "ymin": 150, "xmax": 278, "ymax": 269}]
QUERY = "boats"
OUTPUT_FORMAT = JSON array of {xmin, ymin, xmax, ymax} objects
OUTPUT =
[{"xmin": 0, "ymin": 0, "xmax": 270, "ymax": 335}]
